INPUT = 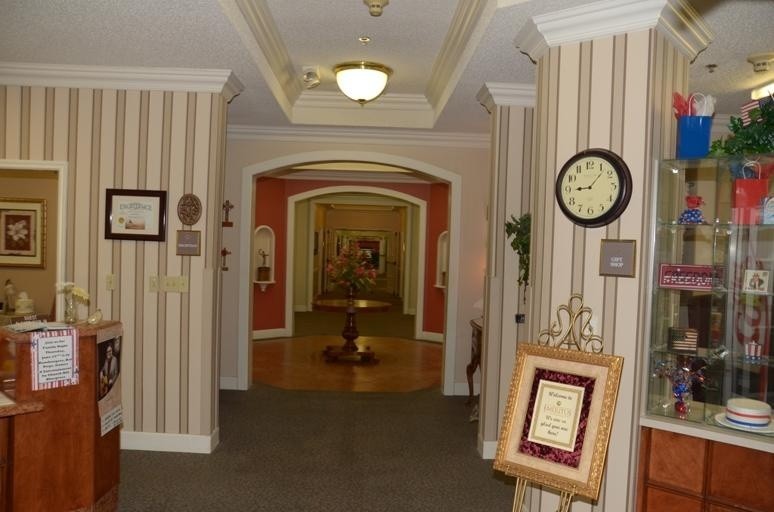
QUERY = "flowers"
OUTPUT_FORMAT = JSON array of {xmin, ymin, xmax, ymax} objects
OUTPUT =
[{"xmin": 323, "ymin": 238, "xmax": 378, "ymax": 297}]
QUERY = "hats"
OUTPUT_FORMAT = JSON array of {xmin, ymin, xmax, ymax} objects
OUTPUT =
[{"xmin": 715, "ymin": 397, "xmax": 774, "ymax": 435}]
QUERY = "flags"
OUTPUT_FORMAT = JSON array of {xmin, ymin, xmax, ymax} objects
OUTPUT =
[{"xmin": 740, "ymin": 96, "xmax": 773, "ymax": 127}]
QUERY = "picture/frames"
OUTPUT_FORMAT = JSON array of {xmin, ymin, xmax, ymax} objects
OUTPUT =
[
  {"xmin": 0, "ymin": 198, "xmax": 47, "ymax": 271},
  {"xmin": 106, "ymin": 189, "xmax": 168, "ymax": 242}
]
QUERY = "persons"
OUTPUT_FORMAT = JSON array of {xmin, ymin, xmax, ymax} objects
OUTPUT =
[
  {"xmin": 749, "ymin": 272, "xmax": 765, "ymax": 291},
  {"xmin": 100, "ymin": 345, "xmax": 118, "ymax": 387}
]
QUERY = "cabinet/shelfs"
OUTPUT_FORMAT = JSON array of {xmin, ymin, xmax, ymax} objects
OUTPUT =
[{"xmin": 646, "ymin": 158, "xmax": 772, "ymax": 451}]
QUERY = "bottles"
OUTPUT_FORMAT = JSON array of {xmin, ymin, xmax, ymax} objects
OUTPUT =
[{"xmin": 2, "ymin": 283, "xmax": 15, "ymax": 315}]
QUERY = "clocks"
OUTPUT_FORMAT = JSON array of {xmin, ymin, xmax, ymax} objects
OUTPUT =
[{"xmin": 555, "ymin": 147, "xmax": 633, "ymax": 230}]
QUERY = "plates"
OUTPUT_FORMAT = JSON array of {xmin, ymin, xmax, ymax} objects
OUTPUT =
[{"xmin": 714, "ymin": 412, "xmax": 774, "ymax": 434}]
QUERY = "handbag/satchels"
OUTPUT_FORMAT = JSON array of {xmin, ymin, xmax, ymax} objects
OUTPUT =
[
  {"xmin": 731, "ymin": 177, "xmax": 769, "ymax": 226},
  {"xmin": 676, "ymin": 117, "xmax": 713, "ymax": 161}
]
